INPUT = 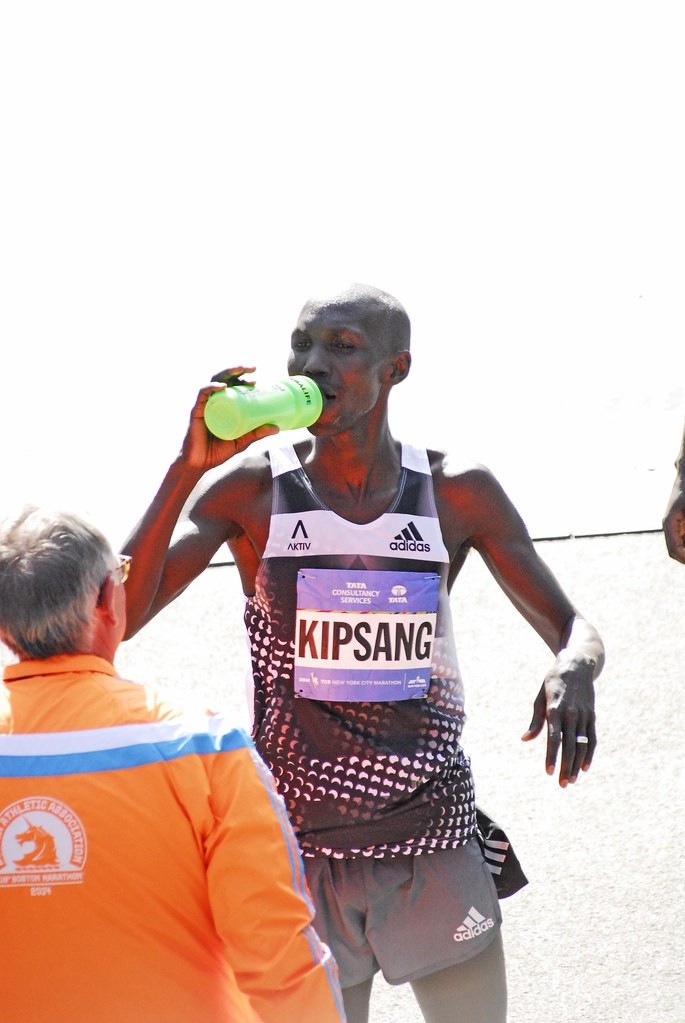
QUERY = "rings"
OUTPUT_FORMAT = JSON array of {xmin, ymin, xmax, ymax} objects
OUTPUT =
[{"xmin": 577, "ymin": 736, "xmax": 589, "ymax": 743}]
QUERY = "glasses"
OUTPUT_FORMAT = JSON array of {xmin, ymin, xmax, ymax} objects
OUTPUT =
[{"xmin": 105, "ymin": 553, "xmax": 133, "ymax": 583}]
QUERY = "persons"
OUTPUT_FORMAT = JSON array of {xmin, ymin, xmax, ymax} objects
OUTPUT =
[
  {"xmin": 663, "ymin": 422, "xmax": 685, "ymax": 565},
  {"xmin": 116, "ymin": 274, "xmax": 603, "ymax": 1023},
  {"xmin": 0, "ymin": 505, "xmax": 347, "ymax": 1023}
]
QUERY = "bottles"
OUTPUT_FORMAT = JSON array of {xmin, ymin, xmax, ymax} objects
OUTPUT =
[{"xmin": 203, "ymin": 374, "xmax": 328, "ymax": 441}]
